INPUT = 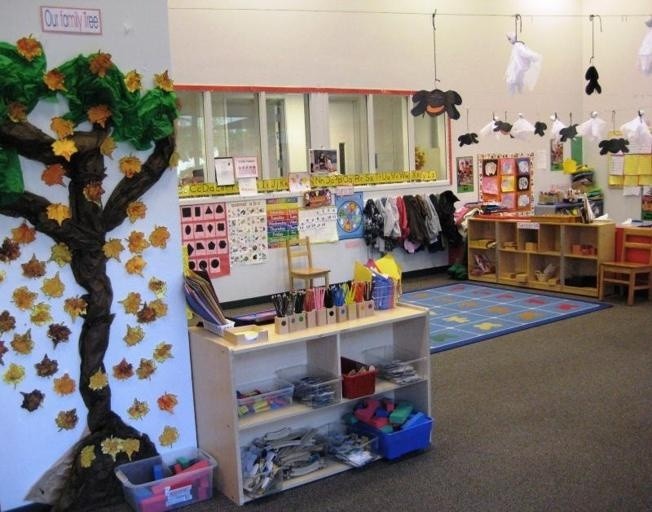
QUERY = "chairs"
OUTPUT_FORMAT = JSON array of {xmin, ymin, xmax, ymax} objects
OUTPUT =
[
  {"xmin": 284, "ymin": 234, "xmax": 333, "ymax": 289},
  {"xmin": 600, "ymin": 226, "xmax": 652, "ymax": 307}
]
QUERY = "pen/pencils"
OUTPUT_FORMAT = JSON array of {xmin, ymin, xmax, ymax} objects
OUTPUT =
[
  {"xmin": 340, "ymin": 280, "xmax": 356, "ymax": 304},
  {"xmin": 305, "ymin": 288, "xmax": 314, "ymax": 312},
  {"xmin": 271, "ymin": 293, "xmax": 289, "ymax": 317},
  {"xmin": 363, "ymin": 281, "xmax": 375, "ymax": 301},
  {"xmin": 335, "ymin": 286, "xmax": 344, "ymax": 306},
  {"xmin": 288, "ymin": 288, "xmax": 296, "ymax": 315},
  {"xmin": 354, "ymin": 281, "xmax": 365, "ymax": 303},
  {"xmin": 295, "ymin": 288, "xmax": 307, "ymax": 313},
  {"xmin": 324, "ymin": 287, "xmax": 335, "ymax": 308},
  {"xmin": 315, "ymin": 287, "xmax": 325, "ymax": 309}
]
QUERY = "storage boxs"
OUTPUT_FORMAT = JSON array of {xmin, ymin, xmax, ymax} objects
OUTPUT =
[{"xmin": 113, "ymin": 448, "xmax": 218, "ymax": 512}]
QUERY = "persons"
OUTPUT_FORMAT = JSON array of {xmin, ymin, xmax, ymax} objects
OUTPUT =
[{"xmin": 313, "ymin": 151, "xmax": 336, "ymax": 173}]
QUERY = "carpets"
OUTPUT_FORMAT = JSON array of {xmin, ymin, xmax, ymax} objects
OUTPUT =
[{"xmin": 400, "ymin": 280, "xmax": 613, "ymax": 354}]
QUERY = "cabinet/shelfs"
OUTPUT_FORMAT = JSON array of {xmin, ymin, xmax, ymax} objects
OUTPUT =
[{"xmin": 464, "ymin": 213, "xmax": 617, "ymax": 302}]
